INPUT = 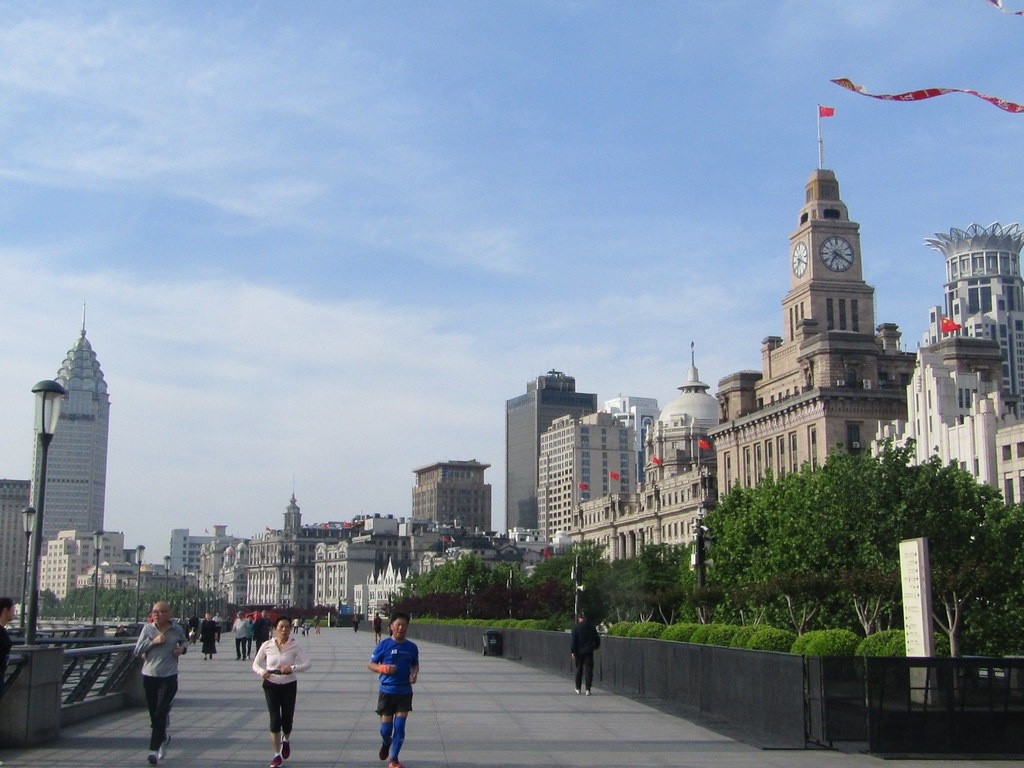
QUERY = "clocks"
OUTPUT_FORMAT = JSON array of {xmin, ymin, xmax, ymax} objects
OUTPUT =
[
  {"xmin": 792, "ymin": 241, "xmax": 808, "ymax": 278},
  {"xmin": 819, "ymin": 236, "xmax": 855, "ymax": 273}
]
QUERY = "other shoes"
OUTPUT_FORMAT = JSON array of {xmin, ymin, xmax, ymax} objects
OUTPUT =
[
  {"xmin": 586, "ymin": 690, "xmax": 592, "ymax": 695},
  {"xmin": 204, "ymin": 658, "xmax": 207, "ymax": 660},
  {"xmin": 236, "ymin": 655, "xmax": 241, "ymax": 660},
  {"xmin": 247, "ymin": 656, "xmax": 249, "ymax": 660},
  {"xmin": 575, "ymin": 688, "xmax": 581, "ymax": 694},
  {"xmin": 210, "ymin": 657, "xmax": 212, "ymax": 659},
  {"xmin": 243, "ymin": 657, "xmax": 245, "ymax": 660}
]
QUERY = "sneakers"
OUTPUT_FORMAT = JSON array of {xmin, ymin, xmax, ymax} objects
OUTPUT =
[
  {"xmin": 388, "ymin": 759, "xmax": 403, "ymax": 768},
  {"xmin": 158, "ymin": 734, "xmax": 170, "ymax": 760},
  {"xmin": 148, "ymin": 750, "xmax": 158, "ymax": 765},
  {"xmin": 270, "ymin": 755, "xmax": 282, "ymax": 768},
  {"xmin": 280, "ymin": 735, "xmax": 290, "ymax": 759},
  {"xmin": 379, "ymin": 737, "xmax": 392, "ymax": 760}
]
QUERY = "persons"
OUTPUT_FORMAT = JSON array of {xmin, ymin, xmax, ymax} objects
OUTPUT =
[
  {"xmin": 252, "ymin": 616, "xmax": 312, "ymax": 768},
  {"xmin": 314, "ymin": 615, "xmax": 321, "ymax": 637},
  {"xmin": 571, "ymin": 610, "xmax": 601, "ymax": 695},
  {"xmin": 353, "ymin": 615, "xmax": 360, "ymax": 633},
  {"xmin": 0, "ymin": 596, "xmax": 16, "ymax": 766},
  {"xmin": 147, "ymin": 610, "xmax": 273, "ymax": 661},
  {"xmin": 134, "ymin": 602, "xmax": 189, "ymax": 765},
  {"xmin": 367, "ymin": 612, "xmax": 420, "ymax": 768}
]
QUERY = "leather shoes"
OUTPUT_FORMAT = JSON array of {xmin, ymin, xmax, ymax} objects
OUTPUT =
[{"xmin": 268, "ymin": 670, "xmax": 292, "ymax": 675}]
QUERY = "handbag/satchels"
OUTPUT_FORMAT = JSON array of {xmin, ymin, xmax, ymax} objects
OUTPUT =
[
  {"xmin": 199, "ymin": 634, "xmax": 204, "ymax": 642},
  {"xmin": 189, "ymin": 628, "xmax": 196, "ymax": 638}
]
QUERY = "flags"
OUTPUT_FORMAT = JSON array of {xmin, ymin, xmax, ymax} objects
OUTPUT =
[
  {"xmin": 344, "ymin": 522, "xmax": 351, "ymax": 527},
  {"xmin": 579, "ymin": 484, "xmax": 588, "ymax": 490},
  {"xmin": 443, "ymin": 536, "xmax": 449, "ymax": 543},
  {"xmin": 544, "ymin": 549, "xmax": 552, "ymax": 558},
  {"xmin": 652, "ymin": 456, "xmax": 661, "ymax": 464},
  {"xmin": 819, "ymin": 106, "xmax": 834, "ymax": 118},
  {"xmin": 698, "ymin": 437, "xmax": 711, "ymax": 448},
  {"xmin": 451, "ymin": 536, "xmax": 456, "ymax": 542},
  {"xmin": 941, "ymin": 317, "xmax": 962, "ymax": 333},
  {"xmin": 611, "ymin": 471, "xmax": 620, "ymax": 480}
]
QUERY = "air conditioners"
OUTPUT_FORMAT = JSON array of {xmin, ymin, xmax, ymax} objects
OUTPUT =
[
  {"xmin": 863, "ymin": 380, "xmax": 872, "ymax": 389},
  {"xmin": 836, "ymin": 380, "xmax": 844, "ymax": 386},
  {"xmin": 853, "ymin": 442, "xmax": 860, "ymax": 448},
  {"xmin": 889, "ymin": 376, "xmax": 895, "ymax": 381}
]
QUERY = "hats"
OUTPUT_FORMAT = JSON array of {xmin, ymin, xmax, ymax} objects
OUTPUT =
[
  {"xmin": 578, "ymin": 611, "xmax": 587, "ymax": 618},
  {"xmin": 117, "ymin": 624, "xmax": 122, "ymax": 628},
  {"xmin": 237, "ymin": 610, "xmax": 244, "ymax": 614}
]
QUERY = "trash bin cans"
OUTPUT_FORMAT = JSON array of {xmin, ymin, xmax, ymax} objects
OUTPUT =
[{"xmin": 483, "ymin": 630, "xmax": 502, "ymax": 656}]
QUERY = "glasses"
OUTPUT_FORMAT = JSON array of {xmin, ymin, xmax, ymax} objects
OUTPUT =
[{"xmin": 152, "ymin": 610, "xmax": 167, "ymax": 615}]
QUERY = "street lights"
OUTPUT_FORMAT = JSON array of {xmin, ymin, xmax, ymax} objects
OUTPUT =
[
  {"xmin": 25, "ymin": 379, "xmax": 68, "ymax": 641},
  {"xmin": 163, "ymin": 555, "xmax": 172, "ymax": 603},
  {"xmin": 195, "ymin": 569, "xmax": 227, "ymax": 618},
  {"xmin": 19, "ymin": 505, "xmax": 37, "ymax": 627},
  {"xmin": 135, "ymin": 545, "xmax": 145, "ymax": 623},
  {"xmin": 91, "ymin": 529, "xmax": 105, "ymax": 625},
  {"xmin": 181, "ymin": 564, "xmax": 187, "ymax": 621}
]
